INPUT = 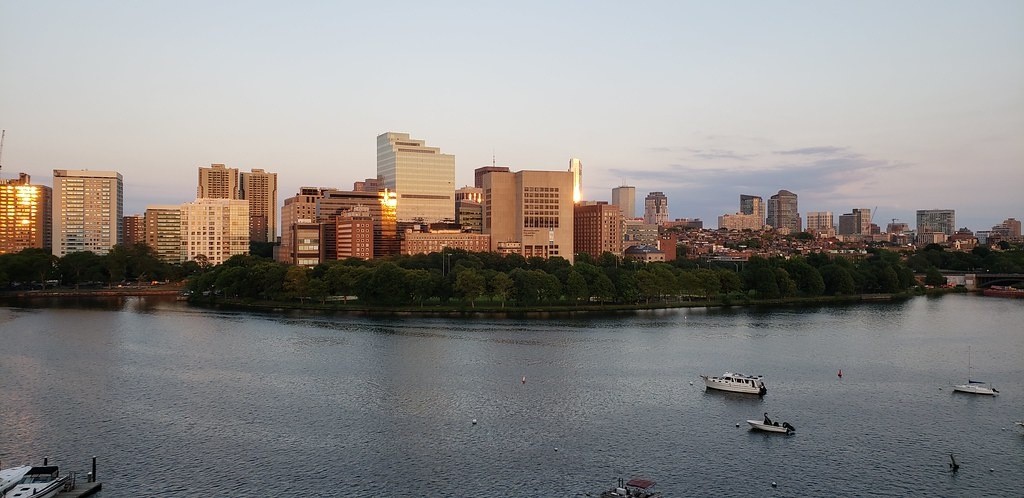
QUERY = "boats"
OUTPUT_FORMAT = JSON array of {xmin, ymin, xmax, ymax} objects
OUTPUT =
[
  {"xmin": 953, "ymin": 384, "xmax": 1000, "ymax": 394},
  {"xmin": 699, "ymin": 371, "xmax": 767, "ymax": 395},
  {"xmin": 574, "ymin": 477, "xmax": 663, "ymax": 498},
  {"xmin": 746, "ymin": 412, "xmax": 796, "ymax": 432},
  {"xmin": 0, "ymin": 457, "xmax": 69, "ymax": 498}
]
